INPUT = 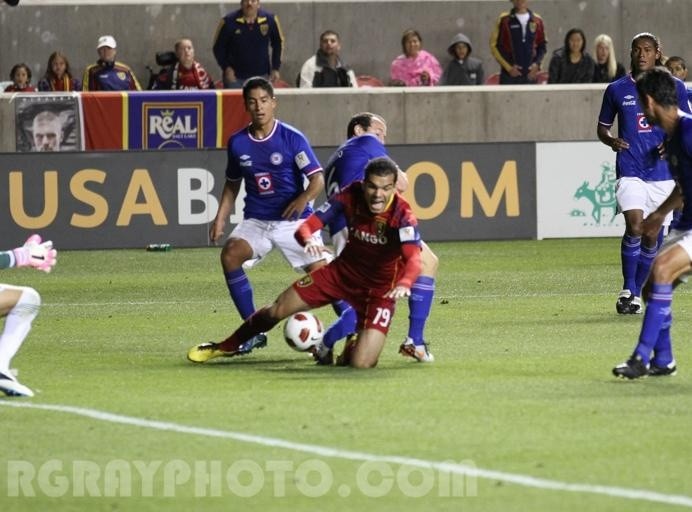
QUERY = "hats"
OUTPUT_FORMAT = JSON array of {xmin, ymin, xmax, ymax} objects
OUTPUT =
[{"xmin": 96, "ymin": 34, "xmax": 116, "ymax": 50}]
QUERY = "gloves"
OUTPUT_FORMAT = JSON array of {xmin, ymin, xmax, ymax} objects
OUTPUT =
[{"xmin": 13, "ymin": 234, "xmax": 57, "ymax": 274}]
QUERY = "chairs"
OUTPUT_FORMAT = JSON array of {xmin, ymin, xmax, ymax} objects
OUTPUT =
[{"xmin": 3, "ymin": 73, "xmax": 691, "ymax": 92}]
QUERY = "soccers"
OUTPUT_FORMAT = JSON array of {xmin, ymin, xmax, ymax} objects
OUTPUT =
[{"xmin": 283, "ymin": 311, "xmax": 325, "ymax": 352}]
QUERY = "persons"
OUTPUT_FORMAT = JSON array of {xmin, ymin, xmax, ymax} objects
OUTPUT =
[
  {"xmin": 596, "ymin": 32, "xmax": 692, "ymax": 315},
  {"xmin": 32, "ymin": 111, "xmax": 61, "ymax": 152},
  {"xmin": 612, "ymin": 69, "xmax": 692, "ymax": 380},
  {"xmin": 313, "ymin": 112, "xmax": 439, "ymax": 363},
  {"xmin": 208, "ymin": 77, "xmax": 351, "ymax": 355},
  {"xmin": 187, "ymin": 157, "xmax": 422, "ymax": 369},
  {"xmin": 0, "ymin": 233, "xmax": 57, "ymax": 397}
]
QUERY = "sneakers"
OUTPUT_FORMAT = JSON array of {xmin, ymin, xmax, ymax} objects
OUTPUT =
[
  {"xmin": 616, "ymin": 289, "xmax": 642, "ymax": 315},
  {"xmin": 613, "ymin": 357, "xmax": 676, "ymax": 379},
  {"xmin": 188, "ymin": 333, "xmax": 268, "ymax": 362},
  {"xmin": 0, "ymin": 371, "xmax": 35, "ymax": 396},
  {"xmin": 399, "ymin": 337, "xmax": 434, "ymax": 363},
  {"xmin": 312, "ymin": 334, "xmax": 358, "ymax": 366}
]
